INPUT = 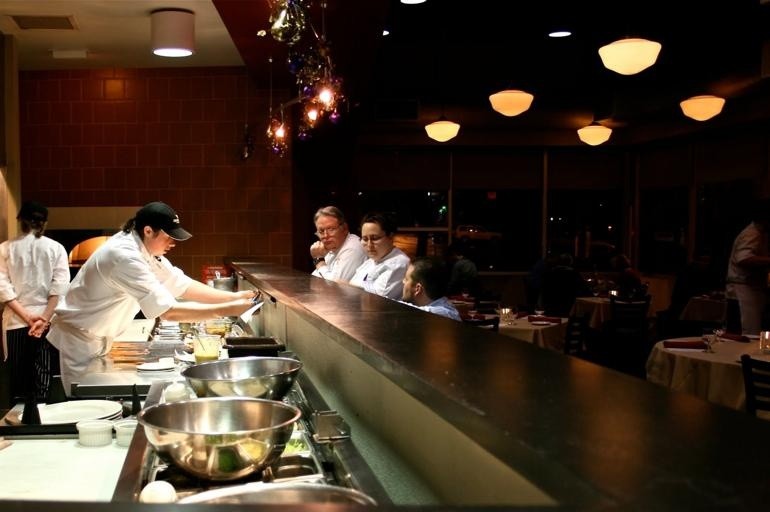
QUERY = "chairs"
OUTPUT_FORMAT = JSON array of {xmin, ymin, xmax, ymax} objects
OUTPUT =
[
  {"xmin": 448, "ymin": 293, "xmax": 570, "ymax": 353},
  {"xmin": 572, "ymin": 272, "xmax": 769, "ymax": 418}
]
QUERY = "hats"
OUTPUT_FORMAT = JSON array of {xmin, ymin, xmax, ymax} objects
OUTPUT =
[
  {"xmin": 135, "ymin": 202, "xmax": 193, "ymax": 242},
  {"xmin": 16, "ymin": 202, "xmax": 48, "ymax": 221}
]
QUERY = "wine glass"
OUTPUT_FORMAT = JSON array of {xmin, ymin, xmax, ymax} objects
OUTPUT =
[
  {"xmin": 701, "ymin": 328, "xmax": 715, "ymax": 353},
  {"xmin": 712, "ymin": 320, "xmax": 727, "ymax": 344},
  {"xmin": 467, "ymin": 305, "xmax": 477, "ymax": 320}
]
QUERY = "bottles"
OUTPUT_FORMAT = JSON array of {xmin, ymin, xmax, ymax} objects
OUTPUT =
[{"xmin": 225, "ymin": 321, "xmax": 233, "ymax": 337}]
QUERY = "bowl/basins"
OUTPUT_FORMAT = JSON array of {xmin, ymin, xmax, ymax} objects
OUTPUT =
[
  {"xmin": 180, "ymin": 355, "xmax": 302, "ymax": 399},
  {"xmin": 177, "ymin": 480, "xmax": 381, "ymax": 505},
  {"xmin": 135, "ymin": 396, "xmax": 300, "ymax": 479}
]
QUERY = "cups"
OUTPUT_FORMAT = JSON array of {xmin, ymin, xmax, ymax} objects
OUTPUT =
[
  {"xmin": 113, "ymin": 420, "xmax": 139, "ymax": 447},
  {"xmin": 75, "ymin": 420, "xmax": 113, "ymax": 447},
  {"xmin": 534, "ymin": 307, "xmax": 545, "ymax": 315},
  {"xmin": 759, "ymin": 331, "xmax": 770, "ymax": 354},
  {"xmin": 584, "ymin": 272, "xmax": 605, "ymax": 300},
  {"xmin": 461, "ymin": 288, "xmax": 469, "ymax": 296},
  {"xmin": 192, "ymin": 335, "xmax": 221, "ymax": 365}
]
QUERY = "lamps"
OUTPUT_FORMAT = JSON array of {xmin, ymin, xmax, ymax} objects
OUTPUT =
[
  {"xmin": 489, "ymin": 89, "xmax": 534, "ymax": 116},
  {"xmin": 426, "ymin": 119, "xmax": 461, "ymax": 141},
  {"xmin": 680, "ymin": 95, "xmax": 725, "ymax": 120},
  {"xmin": 148, "ymin": 7, "xmax": 197, "ymax": 58},
  {"xmin": 233, "ymin": 0, "xmax": 361, "ymax": 166},
  {"xmin": 598, "ymin": 38, "xmax": 664, "ymax": 75}
]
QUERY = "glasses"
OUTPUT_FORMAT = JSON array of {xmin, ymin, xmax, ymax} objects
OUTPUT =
[
  {"xmin": 314, "ymin": 226, "xmax": 343, "ymax": 238},
  {"xmin": 359, "ymin": 233, "xmax": 386, "ymax": 242}
]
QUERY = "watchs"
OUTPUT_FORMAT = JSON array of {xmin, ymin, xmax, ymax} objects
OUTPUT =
[{"xmin": 312, "ymin": 257, "xmax": 325, "ymax": 269}]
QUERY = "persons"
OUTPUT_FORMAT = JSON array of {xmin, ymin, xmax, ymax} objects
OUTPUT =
[
  {"xmin": 0, "ymin": 200, "xmax": 71, "ymax": 398},
  {"xmin": 607, "ymin": 254, "xmax": 642, "ymax": 295},
  {"xmin": 44, "ymin": 201, "xmax": 261, "ymax": 398},
  {"xmin": 542, "ymin": 252, "xmax": 592, "ymax": 317},
  {"xmin": 723, "ymin": 197, "xmax": 770, "ymax": 332},
  {"xmin": 384, "ymin": 254, "xmax": 463, "ymax": 322},
  {"xmin": 335, "ymin": 211, "xmax": 412, "ymax": 302},
  {"xmin": 309, "ymin": 205, "xmax": 368, "ymax": 284}
]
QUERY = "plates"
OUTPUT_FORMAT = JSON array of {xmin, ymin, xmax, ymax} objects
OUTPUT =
[
  {"xmin": 136, "ymin": 362, "xmax": 177, "ymax": 370},
  {"xmin": 741, "ymin": 334, "xmax": 760, "ymax": 339},
  {"xmin": 531, "ymin": 321, "xmax": 550, "ymax": 325},
  {"xmin": 18, "ymin": 399, "xmax": 124, "ymax": 426}
]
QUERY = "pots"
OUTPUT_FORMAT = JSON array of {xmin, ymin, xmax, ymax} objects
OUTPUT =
[{"xmin": 213, "ymin": 276, "xmax": 236, "ymax": 292}]
